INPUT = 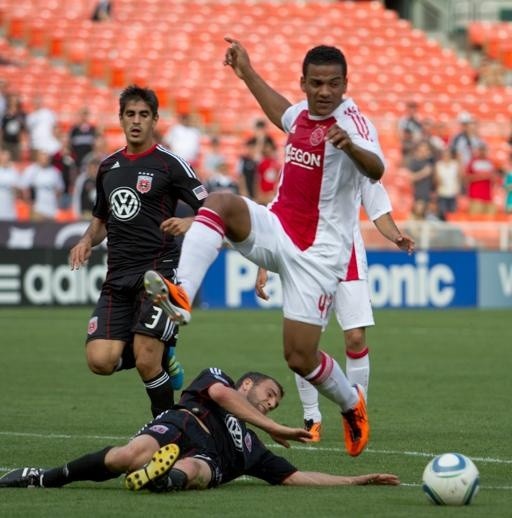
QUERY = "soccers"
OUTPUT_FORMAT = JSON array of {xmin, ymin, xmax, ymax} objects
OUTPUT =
[{"xmin": 420, "ymin": 455, "xmax": 482, "ymax": 508}]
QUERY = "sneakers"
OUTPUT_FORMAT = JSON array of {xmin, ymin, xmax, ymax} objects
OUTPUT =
[
  {"xmin": 340, "ymin": 383, "xmax": 369, "ymax": 457},
  {"xmin": 144, "ymin": 270, "xmax": 192, "ymax": 326},
  {"xmin": 304, "ymin": 419, "xmax": 321, "ymax": 442},
  {"xmin": 168, "ymin": 346, "xmax": 184, "ymax": 390},
  {"xmin": 124, "ymin": 444, "xmax": 180, "ymax": 491},
  {"xmin": 0, "ymin": 467, "xmax": 50, "ymax": 489}
]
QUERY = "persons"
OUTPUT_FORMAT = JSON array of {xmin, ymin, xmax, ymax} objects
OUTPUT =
[
  {"xmin": 139, "ymin": 32, "xmax": 387, "ymax": 458},
  {"xmin": 64, "ymin": 84, "xmax": 212, "ymax": 420},
  {"xmin": 1, "ymin": 364, "xmax": 403, "ymax": 494},
  {"xmin": 291, "ymin": 172, "xmax": 418, "ymax": 446}
]
